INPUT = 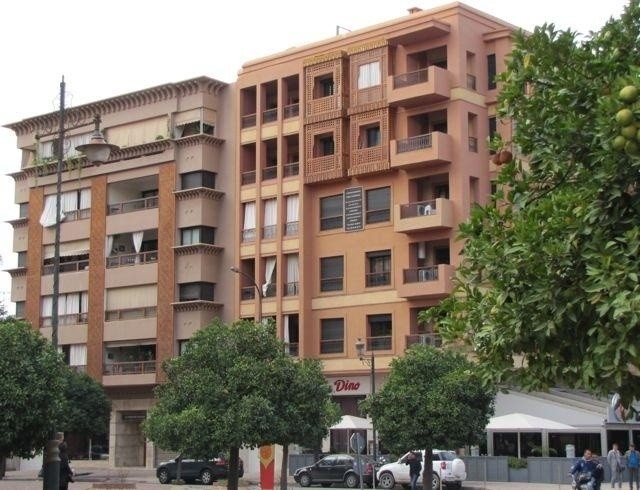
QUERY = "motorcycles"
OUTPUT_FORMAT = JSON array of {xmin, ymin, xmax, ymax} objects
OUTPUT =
[{"xmin": 568, "ymin": 473, "xmax": 592, "ymax": 489}]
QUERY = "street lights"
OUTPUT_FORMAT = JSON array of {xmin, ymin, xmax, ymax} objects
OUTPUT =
[
  {"xmin": 32, "ymin": 71, "xmax": 122, "ymax": 489},
  {"xmin": 229, "ymin": 265, "xmax": 264, "ymax": 327},
  {"xmin": 353, "ymin": 337, "xmax": 378, "ymax": 488}
]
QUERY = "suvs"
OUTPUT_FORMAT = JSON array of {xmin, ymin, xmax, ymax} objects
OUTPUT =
[
  {"xmin": 156, "ymin": 447, "xmax": 243, "ymax": 485},
  {"xmin": 376, "ymin": 448, "xmax": 470, "ymax": 489}
]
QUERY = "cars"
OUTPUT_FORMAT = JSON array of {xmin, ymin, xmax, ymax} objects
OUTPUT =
[
  {"xmin": 292, "ymin": 453, "xmax": 381, "ymax": 488},
  {"xmin": 84, "ymin": 445, "xmax": 108, "ymax": 460}
]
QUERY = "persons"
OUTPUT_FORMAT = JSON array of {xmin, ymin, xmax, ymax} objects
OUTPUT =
[
  {"xmin": 568, "ymin": 448, "xmax": 603, "ymax": 490},
  {"xmin": 607, "ymin": 444, "xmax": 624, "ymax": 490},
  {"xmin": 57, "ymin": 442, "xmax": 76, "ymax": 489},
  {"xmin": 623, "ymin": 444, "xmax": 640, "ymax": 490},
  {"xmin": 406, "ymin": 452, "xmax": 421, "ymax": 490},
  {"xmin": 591, "ymin": 452, "xmax": 604, "ymax": 490}
]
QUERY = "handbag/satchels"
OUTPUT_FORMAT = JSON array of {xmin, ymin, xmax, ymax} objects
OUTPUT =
[{"xmin": 616, "ymin": 464, "xmax": 626, "ymax": 473}]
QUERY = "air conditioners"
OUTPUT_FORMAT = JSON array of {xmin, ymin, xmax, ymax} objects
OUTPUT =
[
  {"xmin": 417, "ymin": 205, "xmax": 425, "ymax": 216},
  {"xmin": 418, "ymin": 269, "xmax": 433, "ymax": 281},
  {"xmin": 419, "ymin": 334, "xmax": 436, "ymax": 348}
]
